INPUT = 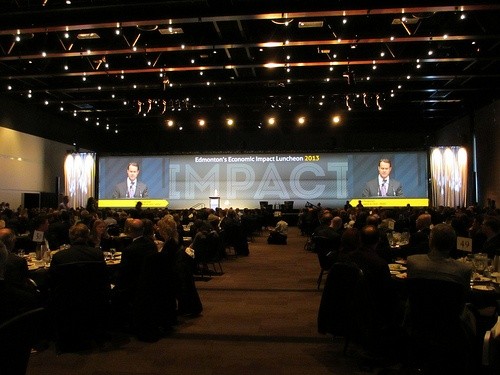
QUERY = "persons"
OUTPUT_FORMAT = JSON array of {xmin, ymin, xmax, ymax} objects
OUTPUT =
[
  {"xmin": 1, "ymin": 195, "xmax": 288, "ymax": 357},
  {"xmin": 361, "ymin": 159, "xmax": 406, "ymax": 197},
  {"xmin": 299, "ymin": 202, "xmax": 500, "ymax": 375},
  {"xmin": 114, "ymin": 162, "xmax": 149, "ymax": 199}
]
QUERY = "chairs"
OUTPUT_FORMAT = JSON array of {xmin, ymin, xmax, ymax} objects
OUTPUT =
[
  {"xmin": 115, "ymin": 214, "xmax": 275, "ymax": 343},
  {"xmin": 0, "ymin": 306, "xmax": 45, "ymax": 375},
  {"xmin": 48, "ymin": 261, "xmax": 107, "ymax": 353},
  {"xmin": 299, "ymin": 214, "xmax": 481, "ymax": 375}
]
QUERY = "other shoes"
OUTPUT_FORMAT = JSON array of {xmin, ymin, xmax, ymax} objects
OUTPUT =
[{"xmin": 27, "ymin": 341, "xmax": 49, "ymax": 353}]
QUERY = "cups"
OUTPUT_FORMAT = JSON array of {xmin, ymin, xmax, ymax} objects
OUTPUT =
[
  {"xmin": 18, "ymin": 249, "xmax": 25, "ymax": 257},
  {"xmin": 36, "ymin": 243, "xmax": 48, "ymax": 259},
  {"xmin": 474, "ymin": 253, "xmax": 487, "ymax": 270},
  {"xmin": 59, "ymin": 244, "xmax": 70, "ymax": 251}
]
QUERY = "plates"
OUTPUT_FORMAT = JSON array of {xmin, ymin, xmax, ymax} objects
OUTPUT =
[
  {"xmin": 103, "ymin": 251, "xmax": 121, "ymax": 256},
  {"xmin": 471, "ymin": 271, "xmax": 500, "ymax": 291},
  {"xmin": 387, "ymin": 259, "xmax": 407, "ymax": 279}
]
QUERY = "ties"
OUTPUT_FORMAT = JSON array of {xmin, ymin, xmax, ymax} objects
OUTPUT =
[
  {"xmin": 380, "ymin": 180, "xmax": 387, "ymax": 196},
  {"xmin": 128, "ymin": 181, "xmax": 135, "ymax": 199}
]
